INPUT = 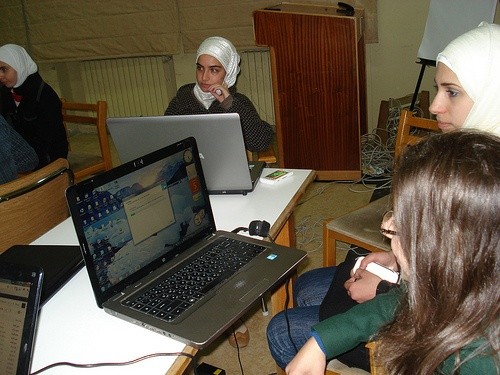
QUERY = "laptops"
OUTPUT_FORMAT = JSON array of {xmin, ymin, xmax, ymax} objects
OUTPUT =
[
  {"xmin": 106, "ymin": 113, "xmax": 266, "ymax": 195},
  {"xmin": 0, "ymin": 261, "xmax": 45, "ymax": 375},
  {"xmin": 65, "ymin": 136, "xmax": 309, "ymax": 351}
]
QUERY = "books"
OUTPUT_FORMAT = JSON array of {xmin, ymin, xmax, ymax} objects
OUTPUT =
[{"xmin": 260, "ymin": 170, "xmax": 294, "ymax": 184}]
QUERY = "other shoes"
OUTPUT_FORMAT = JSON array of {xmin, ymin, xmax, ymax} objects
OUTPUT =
[{"xmin": 227, "ymin": 318, "xmax": 249, "ymax": 348}]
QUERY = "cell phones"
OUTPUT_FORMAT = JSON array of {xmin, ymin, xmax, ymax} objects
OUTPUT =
[{"xmin": 259, "ymin": 169, "xmax": 293, "ymax": 185}]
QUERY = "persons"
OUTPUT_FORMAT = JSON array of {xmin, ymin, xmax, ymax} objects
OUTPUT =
[
  {"xmin": 163, "ymin": 36, "xmax": 272, "ymax": 152},
  {"xmin": 283, "ymin": 131, "xmax": 500, "ymax": 375},
  {"xmin": 0, "ymin": 116, "xmax": 37, "ymax": 184},
  {"xmin": 0, "ymin": 44, "xmax": 69, "ymax": 169},
  {"xmin": 267, "ymin": 21, "xmax": 500, "ymax": 375}
]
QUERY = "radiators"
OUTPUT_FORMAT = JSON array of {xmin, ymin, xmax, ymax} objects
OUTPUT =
[
  {"xmin": 79, "ymin": 58, "xmax": 170, "ymax": 118},
  {"xmin": 240, "ymin": 49, "xmax": 275, "ymax": 126}
]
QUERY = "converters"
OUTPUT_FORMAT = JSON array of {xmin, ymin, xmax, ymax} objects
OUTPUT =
[{"xmin": 194, "ymin": 362, "xmax": 226, "ymax": 375}]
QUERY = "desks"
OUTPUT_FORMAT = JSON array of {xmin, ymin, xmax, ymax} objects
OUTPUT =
[{"xmin": 29, "ymin": 168, "xmax": 317, "ymax": 375}]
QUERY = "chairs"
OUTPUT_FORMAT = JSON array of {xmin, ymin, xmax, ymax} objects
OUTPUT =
[
  {"xmin": 323, "ymin": 110, "xmax": 442, "ymax": 268},
  {"xmin": 62, "ymin": 101, "xmax": 112, "ymax": 182},
  {"xmin": 0, "ymin": 158, "xmax": 74, "ymax": 253}
]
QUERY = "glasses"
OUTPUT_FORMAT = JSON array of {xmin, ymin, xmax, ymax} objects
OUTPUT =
[{"xmin": 380, "ymin": 211, "xmax": 401, "ymax": 239}]
